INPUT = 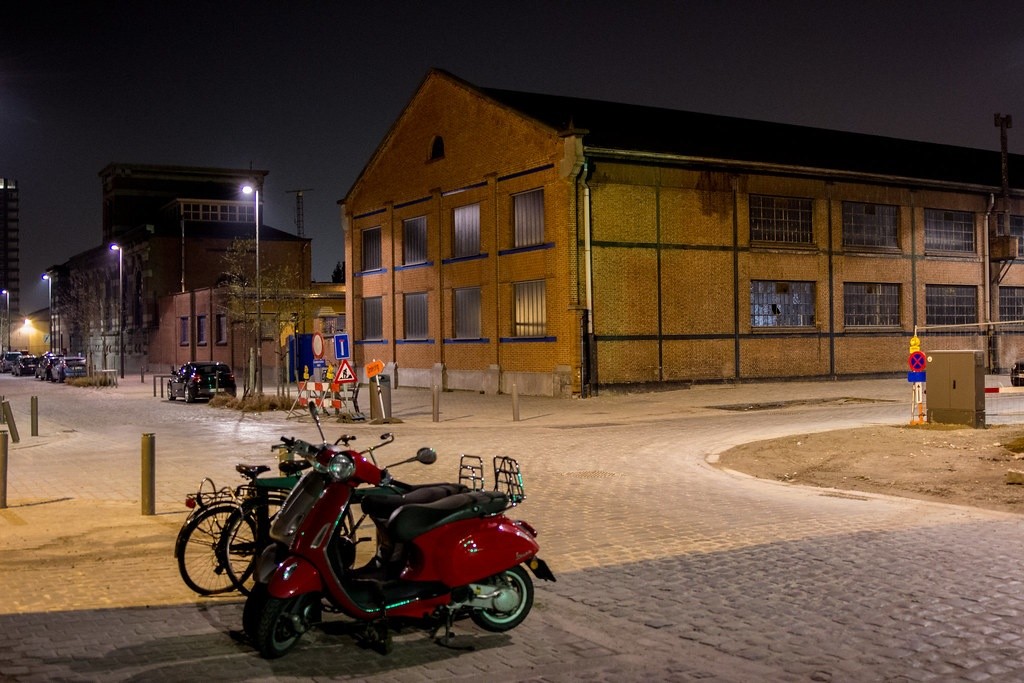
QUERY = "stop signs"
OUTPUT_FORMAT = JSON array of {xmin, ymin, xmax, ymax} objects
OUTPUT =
[{"xmin": 366, "ymin": 359, "xmax": 385, "ymax": 379}]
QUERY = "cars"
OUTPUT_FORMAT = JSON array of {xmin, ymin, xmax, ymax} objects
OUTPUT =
[
  {"xmin": 0, "ymin": 351, "xmax": 86, "ymax": 384},
  {"xmin": 167, "ymin": 361, "xmax": 237, "ymax": 402}
]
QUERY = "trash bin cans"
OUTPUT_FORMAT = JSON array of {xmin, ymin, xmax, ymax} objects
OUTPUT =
[{"xmin": 370, "ymin": 374, "xmax": 392, "ymax": 421}]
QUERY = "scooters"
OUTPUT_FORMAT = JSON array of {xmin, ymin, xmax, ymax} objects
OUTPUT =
[{"xmin": 242, "ymin": 400, "xmax": 557, "ymax": 657}]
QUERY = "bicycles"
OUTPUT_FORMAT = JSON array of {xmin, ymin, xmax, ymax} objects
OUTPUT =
[{"xmin": 174, "ymin": 433, "xmax": 395, "ymax": 596}]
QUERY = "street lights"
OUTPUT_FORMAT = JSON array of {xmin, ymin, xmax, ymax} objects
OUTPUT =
[
  {"xmin": 43, "ymin": 274, "xmax": 55, "ymax": 351},
  {"xmin": 241, "ymin": 182, "xmax": 267, "ymax": 401},
  {"xmin": 21, "ymin": 319, "xmax": 35, "ymax": 354},
  {"xmin": 2, "ymin": 289, "xmax": 11, "ymax": 351},
  {"xmin": 110, "ymin": 244, "xmax": 126, "ymax": 378}
]
